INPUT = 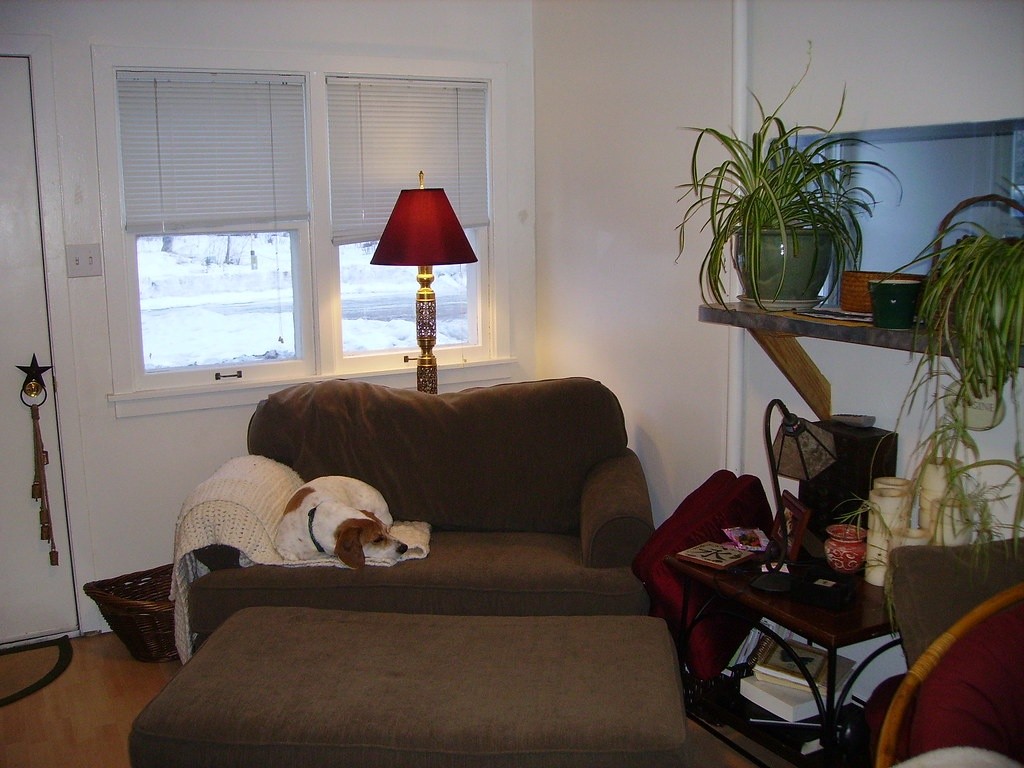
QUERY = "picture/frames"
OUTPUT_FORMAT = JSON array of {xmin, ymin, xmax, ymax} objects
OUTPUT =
[{"xmin": 769, "ymin": 489, "xmax": 827, "ymax": 564}]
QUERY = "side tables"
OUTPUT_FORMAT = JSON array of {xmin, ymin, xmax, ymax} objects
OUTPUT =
[{"xmin": 665, "ymin": 556, "xmax": 904, "ymax": 768}]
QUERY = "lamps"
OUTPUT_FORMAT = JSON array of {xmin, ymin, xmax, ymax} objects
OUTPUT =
[
  {"xmin": 369, "ymin": 171, "xmax": 481, "ymax": 397},
  {"xmin": 750, "ymin": 399, "xmax": 835, "ymax": 596}
]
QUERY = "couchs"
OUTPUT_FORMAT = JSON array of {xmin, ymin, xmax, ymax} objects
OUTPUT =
[{"xmin": 185, "ymin": 377, "xmax": 656, "ymax": 653}]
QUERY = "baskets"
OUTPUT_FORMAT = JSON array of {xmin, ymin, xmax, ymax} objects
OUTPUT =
[{"xmin": 83, "ymin": 562, "xmax": 179, "ymax": 663}]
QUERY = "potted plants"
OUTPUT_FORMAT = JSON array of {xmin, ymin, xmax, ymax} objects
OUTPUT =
[
  {"xmin": 864, "ymin": 218, "xmax": 1024, "ymax": 557},
  {"xmin": 674, "ymin": 41, "xmax": 902, "ymax": 318}
]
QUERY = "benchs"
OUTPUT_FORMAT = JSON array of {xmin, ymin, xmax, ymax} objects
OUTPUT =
[{"xmin": 122, "ymin": 604, "xmax": 698, "ymax": 768}]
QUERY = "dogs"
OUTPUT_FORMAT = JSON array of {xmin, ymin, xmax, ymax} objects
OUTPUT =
[{"xmin": 274, "ymin": 475, "xmax": 409, "ymax": 569}]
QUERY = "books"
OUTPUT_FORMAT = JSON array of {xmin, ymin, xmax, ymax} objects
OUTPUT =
[
  {"xmin": 676, "ymin": 541, "xmax": 755, "ymax": 570},
  {"xmin": 739, "ymin": 638, "xmax": 856, "ymax": 724}
]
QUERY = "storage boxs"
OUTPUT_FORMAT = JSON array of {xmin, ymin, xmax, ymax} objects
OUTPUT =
[{"xmin": 736, "ymin": 676, "xmax": 854, "ymax": 722}]
